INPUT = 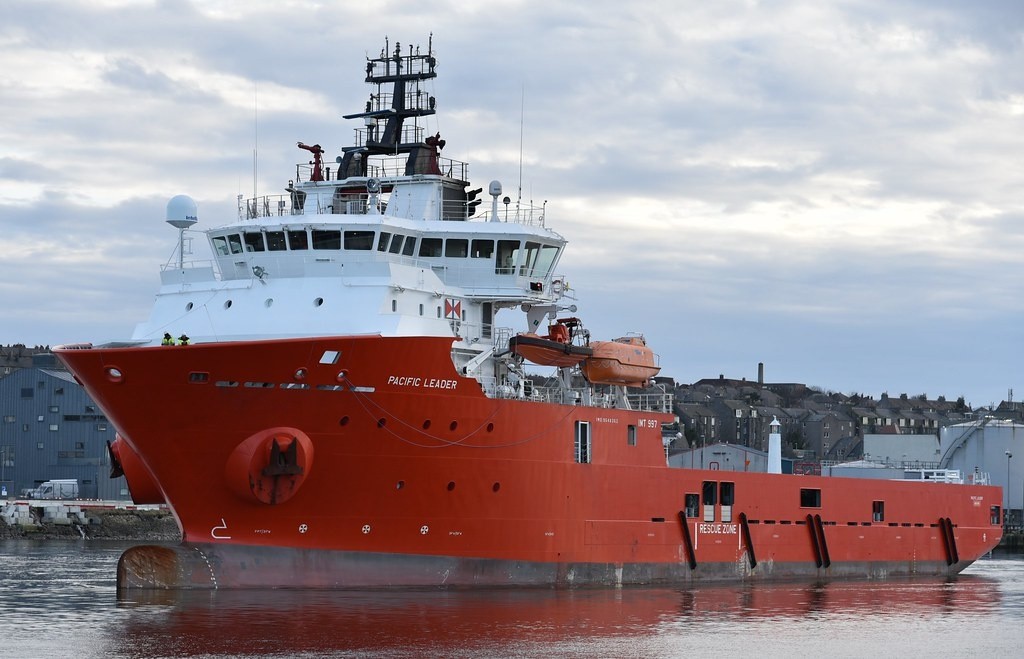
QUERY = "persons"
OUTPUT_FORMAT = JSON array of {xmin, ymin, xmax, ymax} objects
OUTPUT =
[
  {"xmin": 161, "ymin": 331, "xmax": 175, "ymax": 346},
  {"xmin": 178, "ymin": 332, "xmax": 190, "ymax": 345}
]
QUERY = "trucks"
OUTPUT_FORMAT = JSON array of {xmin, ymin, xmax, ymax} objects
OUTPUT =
[{"xmin": 32, "ymin": 479, "xmax": 86, "ymax": 500}]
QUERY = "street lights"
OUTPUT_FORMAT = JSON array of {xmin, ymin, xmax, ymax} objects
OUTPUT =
[
  {"xmin": 700, "ymin": 434, "xmax": 705, "ymax": 469},
  {"xmin": 795, "ymin": 443, "xmax": 798, "ymax": 450},
  {"xmin": 1005, "ymin": 449, "xmax": 1013, "ymax": 524},
  {"xmin": 691, "ymin": 440, "xmax": 696, "ymax": 468}
]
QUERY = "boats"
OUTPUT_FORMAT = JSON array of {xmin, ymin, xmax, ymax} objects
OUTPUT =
[
  {"xmin": 509, "ymin": 316, "xmax": 593, "ymax": 368},
  {"xmin": 50, "ymin": 31, "xmax": 1005, "ymax": 590},
  {"xmin": 579, "ymin": 331, "xmax": 662, "ymax": 389}
]
